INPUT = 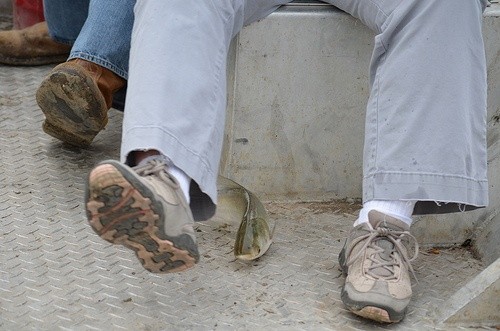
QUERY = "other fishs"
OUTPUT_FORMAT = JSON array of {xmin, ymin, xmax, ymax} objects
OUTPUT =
[{"xmin": 196, "ymin": 185, "xmax": 272, "ymax": 262}]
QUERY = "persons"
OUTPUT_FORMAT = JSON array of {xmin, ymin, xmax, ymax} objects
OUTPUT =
[
  {"xmin": 0, "ymin": 0, "xmax": 137, "ymax": 150},
  {"xmin": 82, "ymin": 0, "xmax": 490, "ymax": 324}
]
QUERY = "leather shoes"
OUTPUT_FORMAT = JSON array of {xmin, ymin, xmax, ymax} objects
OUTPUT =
[
  {"xmin": 34, "ymin": 59, "xmax": 119, "ymax": 148},
  {"xmin": 0, "ymin": 23, "xmax": 73, "ymax": 66}
]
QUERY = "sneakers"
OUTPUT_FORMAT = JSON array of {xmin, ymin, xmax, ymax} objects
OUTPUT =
[
  {"xmin": 83, "ymin": 157, "xmax": 200, "ymax": 274},
  {"xmin": 337, "ymin": 210, "xmax": 414, "ymax": 323}
]
